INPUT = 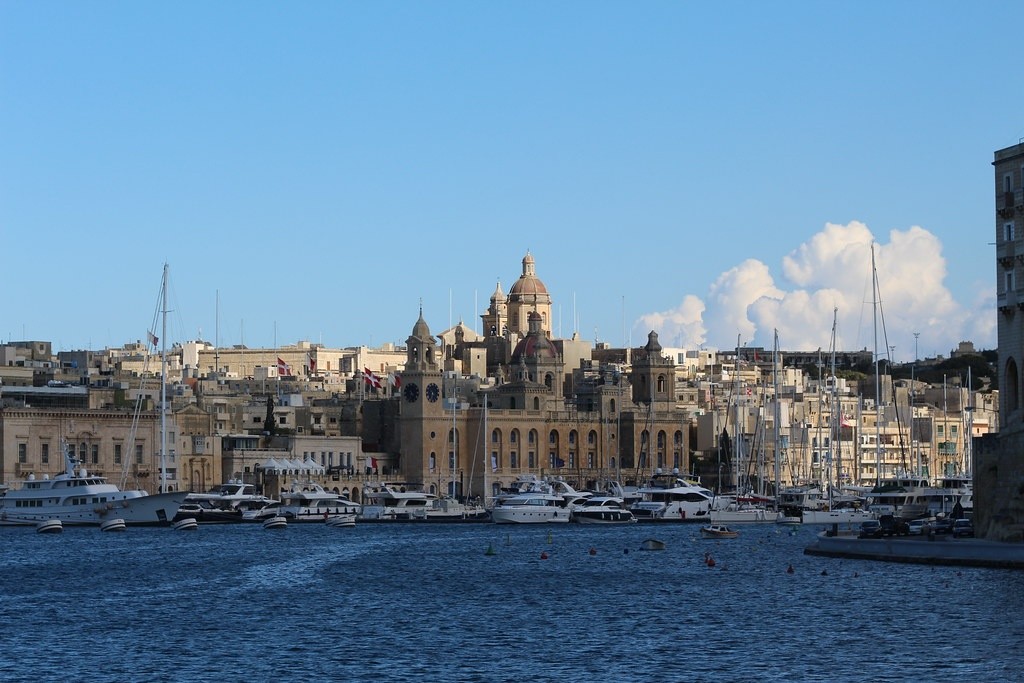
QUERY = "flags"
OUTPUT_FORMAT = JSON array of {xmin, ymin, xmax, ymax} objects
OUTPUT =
[
  {"xmin": 840, "ymin": 409, "xmax": 852, "ymax": 428},
  {"xmin": 307, "ymin": 353, "xmax": 315, "ymax": 372},
  {"xmin": 754, "ymin": 351, "xmax": 765, "ymax": 362},
  {"xmin": 388, "ymin": 371, "xmax": 401, "ymax": 389},
  {"xmin": 365, "ymin": 454, "xmax": 378, "ymax": 468},
  {"xmin": 148, "ymin": 331, "xmax": 158, "ymax": 346},
  {"xmin": 278, "ymin": 357, "xmax": 291, "ymax": 377},
  {"xmin": 362, "ymin": 367, "xmax": 382, "ymax": 389},
  {"xmin": 745, "ymin": 386, "xmax": 752, "ymax": 397}
]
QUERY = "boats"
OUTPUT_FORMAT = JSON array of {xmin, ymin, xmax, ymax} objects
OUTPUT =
[
  {"xmin": 552, "ymin": 482, "xmax": 639, "ymax": 527},
  {"xmin": 281, "ymin": 482, "xmax": 362, "ymax": 528},
  {"xmin": 656, "ymin": 238, "xmax": 975, "ymax": 525},
  {"xmin": 2, "ymin": 436, "xmax": 188, "ymax": 525},
  {"xmin": 428, "ymin": 495, "xmax": 489, "ymax": 521},
  {"xmin": 355, "ymin": 480, "xmax": 433, "ymax": 522},
  {"xmin": 492, "ymin": 490, "xmax": 570, "ymax": 522},
  {"xmin": 321, "ymin": 513, "xmax": 358, "ymax": 526},
  {"xmin": 213, "ymin": 480, "xmax": 282, "ymax": 520},
  {"xmin": 175, "ymin": 478, "xmax": 243, "ymax": 520}
]
